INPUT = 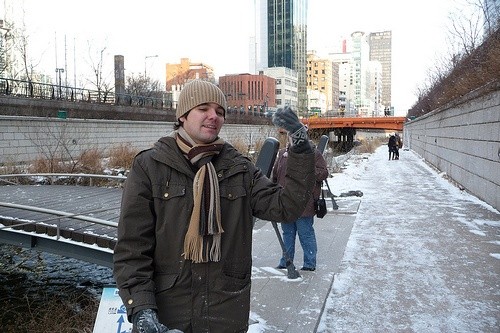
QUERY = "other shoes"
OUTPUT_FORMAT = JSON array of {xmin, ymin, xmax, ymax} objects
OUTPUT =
[
  {"xmin": 389, "ymin": 159, "xmax": 390, "ymax": 160},
  {"xmin": 301, "ymin": 267, "xmax": 316, "ymax": 271},
  {"xmin": 395, "ymin": 157, "xmax": 399, "ymax": 160},
  {"xmin": 392, "ymin": 158, "xmax": 394, "ymax": 160},
  {"xmin": 275, "ymin": 266, "xmax": 287, "ymax": 270}
]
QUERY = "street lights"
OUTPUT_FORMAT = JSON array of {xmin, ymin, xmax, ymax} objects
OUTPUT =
[
  {"xmin": 98, "ymin": 46, "xmax": 107, "ymax": 91},
  {"xmin": 55, "ymin": 68, "xmax": 64, "ymax": 99},
  {"xmin": 143, "ymin": 54, "xmax": 158, "ymax": 90}
]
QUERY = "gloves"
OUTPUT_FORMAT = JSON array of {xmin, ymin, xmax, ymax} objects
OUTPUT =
[
  {"xmin": 272, "ymin": 106, "xmax": 311, "ymax": 145},
  {"xmin": 132, "ymin": 310, "xmax": 168, "ymax": 333}
]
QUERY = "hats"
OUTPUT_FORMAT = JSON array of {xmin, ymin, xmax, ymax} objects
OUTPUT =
[{"xmin": 175, "ymin": 81, "xmax": 227, "ymax": 119}]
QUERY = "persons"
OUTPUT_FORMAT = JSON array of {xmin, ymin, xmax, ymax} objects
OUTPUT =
[
  {"xmin": 388, "ymin": 131, "xmax": 401, "ymax": 161},
  {"xmin": 273, "ymin": 124, "xmax": 329, "ymax": 271},
  {"xmin": 384, "ymin": 108, "xmax": 391, "ymax": 117},
  {"xmin": 113, "ymin": 80, "xmax": 315, "ymax": 333}
]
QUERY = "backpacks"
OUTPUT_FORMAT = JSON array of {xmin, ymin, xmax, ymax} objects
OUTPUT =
[{"xmin": 399, "ymin": 141, "xmax": 403, "ymax": 148}]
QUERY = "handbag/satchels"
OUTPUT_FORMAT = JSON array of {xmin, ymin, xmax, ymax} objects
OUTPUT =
[{"xmin": 315, "ymin": 199, "xmax": 328, "ymax": 218}]
